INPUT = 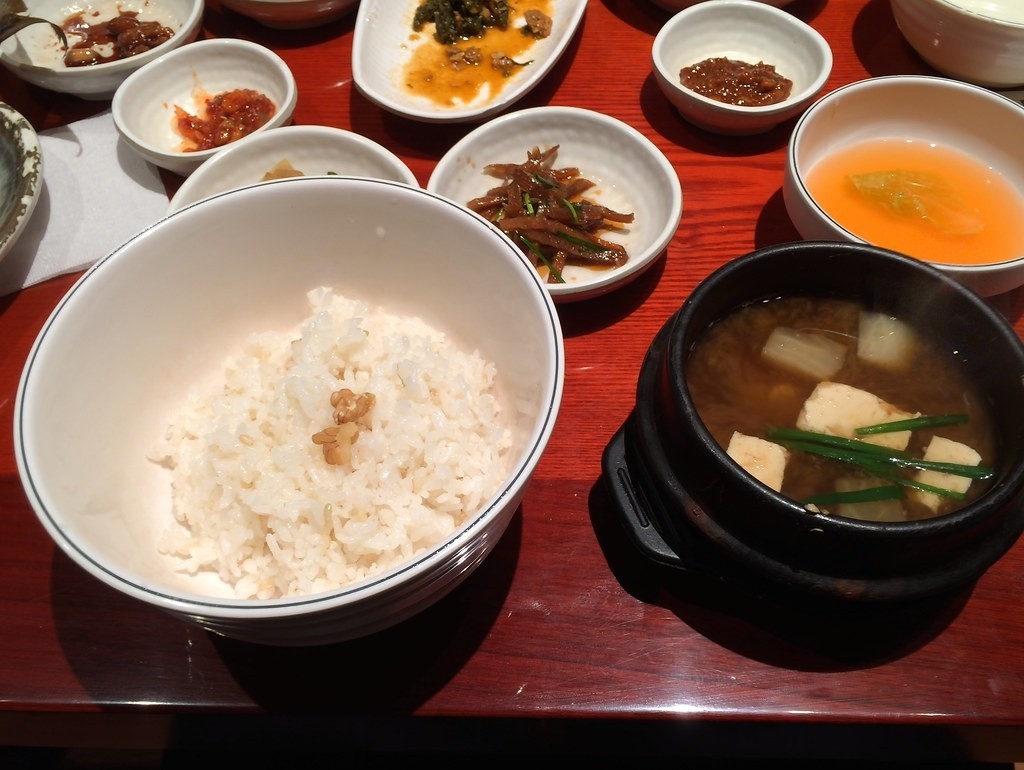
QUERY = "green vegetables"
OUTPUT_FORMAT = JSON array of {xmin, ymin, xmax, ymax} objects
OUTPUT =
[
  {"xmin": 494, "ymin": 174, "xmax": 612, "ymax": 283},
  {"xmin": 761, "ymin": 413, "xmax": 1000, "ymax": 509}
]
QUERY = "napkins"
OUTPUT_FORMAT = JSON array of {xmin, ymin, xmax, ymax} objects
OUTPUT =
[{"xmin": 0, "ymin": 110, "xmax": 169, "ymax": 296}]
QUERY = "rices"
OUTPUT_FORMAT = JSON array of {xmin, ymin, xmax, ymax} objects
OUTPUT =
[{"xmin": 142, "ymin": 285, "xmax": 510, "ymax": 601}]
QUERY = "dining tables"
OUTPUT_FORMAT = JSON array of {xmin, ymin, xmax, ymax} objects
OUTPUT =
[{"xmin": 0, "ymin": 0, "xmax": 1024, "ymax": 770}]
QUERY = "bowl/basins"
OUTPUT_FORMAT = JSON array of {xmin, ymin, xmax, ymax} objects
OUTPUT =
[
  {"xmin": 891, "ymin": 1, "xmax": 1024, "ymax": 89},
  {"xmin": 786, "ymin": 72, "xmax": 1024, "ymax": 299},
  {"xmin": 14, "ymin": 178, "xmax": 565, "ymax": 650},
  {"xmin": 112, "ymin": 40, "xmax": 297, "ymax": 178},
  {"xmin": 2, "ymin": 1, "xmax": 203, "ymax": 104},
  {"xmin": 652, "ymin": 2, "xmax": 833, "ymax": 136},
  {"xmin": 600, "ymin": 246, "xmax": 1024, "ymax": 666},
  {"xmin": 1, "ymin": 107, "xmax": 43, "ymax": 275}
]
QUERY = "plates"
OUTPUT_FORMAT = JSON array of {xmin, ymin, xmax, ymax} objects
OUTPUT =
[
  {"xmin": 351, "ymin": 1, "xmax": 589, "ymax": 124},
  {"xmin": 427, "ymin": 107, "xmax": 682, "ymax": 307},
  {"xmin": 166, "ymin": 127, "xmax": 422, "ymax": 232}
]
